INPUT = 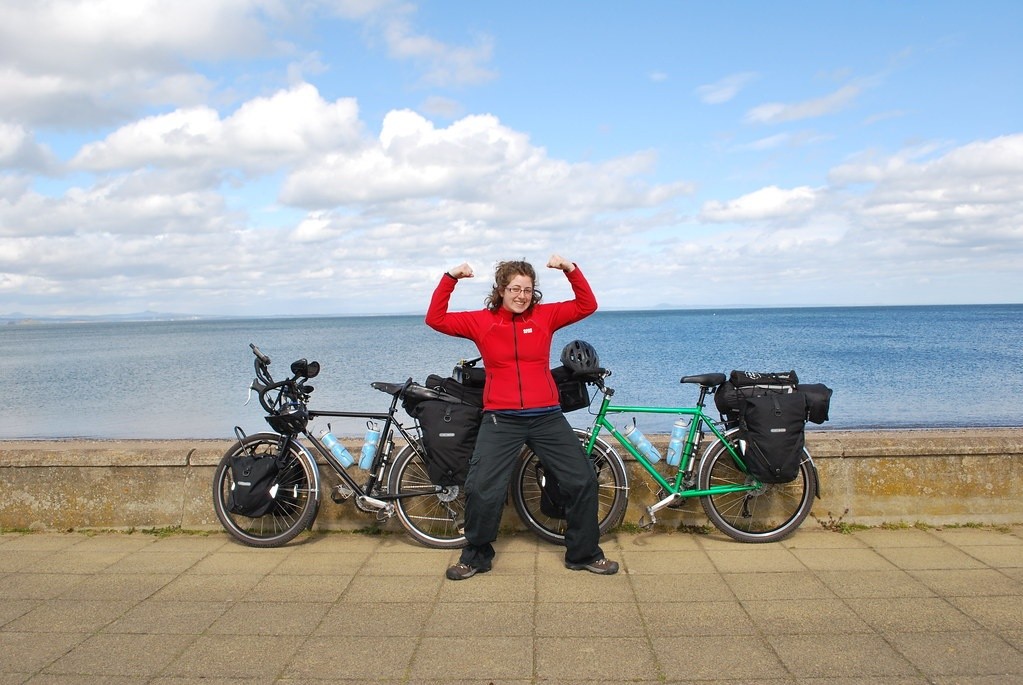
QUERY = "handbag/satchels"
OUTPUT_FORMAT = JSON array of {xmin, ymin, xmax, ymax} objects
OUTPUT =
[
  {"xmin": 714, "ymin": 369, "xmax": 832, "ymax": 483},
  {"xmin": 402, "ymin": 360, "xmax": 486, "ymax": 484},
  {"xmin": 228, "ymin": 453, "xmax": 304, "ymax": 518}
]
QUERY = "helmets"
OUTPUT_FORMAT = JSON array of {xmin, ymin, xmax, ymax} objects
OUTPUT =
[
  {"xmin": 561, "ymin": 340, "xmax": 599, "ymax": 377},
  {"xmin": 264, "ymin": 403, "xmax": 310, "ymax": 434}
]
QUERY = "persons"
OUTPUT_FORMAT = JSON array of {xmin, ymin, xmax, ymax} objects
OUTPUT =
[{"xmin": 426, "ymin": 255, "xmax": 619, "ymax": 581}]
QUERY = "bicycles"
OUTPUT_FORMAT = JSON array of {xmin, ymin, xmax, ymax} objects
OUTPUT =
[
  {"xmin": 211, "ymin": 360, "xmax": 472, "ymax": 550},
  {"xmin": 510, "ymin": 367, "xmax": 821, "ymax": 547}
]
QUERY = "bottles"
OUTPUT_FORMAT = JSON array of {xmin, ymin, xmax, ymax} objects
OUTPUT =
[
  {"xmin": 319, "ymin": 423, "xmax": 354, "ymax": 470},
  {"xmin": 624, "ymin": 424, "xmax": 662, "ymax": 464},
  {"xmin": 359, "ymin": 420, "xmax": 380, "ymax": 471},
  {"xmin": 666, "ymin": 418, "xmax": 686, "ymax": 467}
]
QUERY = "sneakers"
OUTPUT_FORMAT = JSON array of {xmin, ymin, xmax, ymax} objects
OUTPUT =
[
  {"xmin": 446, "ymin": 562, "xmax": 491, "ymax": 580},
  {"xmin": 565, "ymin": 557, "xmax": 619, "ymax": 574}
]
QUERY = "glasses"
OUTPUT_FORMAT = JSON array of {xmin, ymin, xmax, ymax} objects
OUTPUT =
[{"xmin": 504, "ymin": 287, "xmax": 535, "ymax": 295}]
